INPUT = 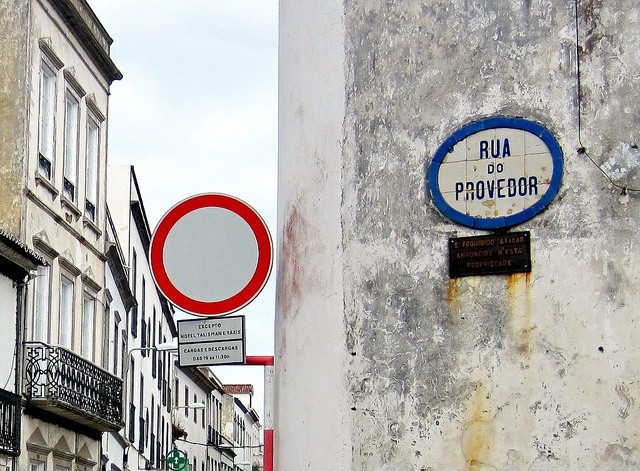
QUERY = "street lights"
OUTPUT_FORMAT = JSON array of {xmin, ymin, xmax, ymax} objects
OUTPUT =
[
  {"xmin": 233, "ymin": 461, "xmax": 251, "ymax": 470},
  {"xmin": 203, "ymin": 443, "xmax": 232, "ymax": 470},
  {"xmin": 170, "ymin": 402, "xmax": 206, "ymax": 471},
  {"xmin": 126, "ymin": 341, "xmax": 178, "ymax": 469}
]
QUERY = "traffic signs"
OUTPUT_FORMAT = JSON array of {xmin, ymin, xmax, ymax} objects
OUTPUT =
[
  {"xmin": 178, "ymin": 339, "xmax": 245, "ymax": 367},
  {"xmin": 178, "ymin": 315, "xmax": 246, "ymax": 342}
]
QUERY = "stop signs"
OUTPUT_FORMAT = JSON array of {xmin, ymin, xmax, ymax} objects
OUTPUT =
[{"xmin": 149, "ymin": 192, "xmax": 274, "ymax": 318}]
